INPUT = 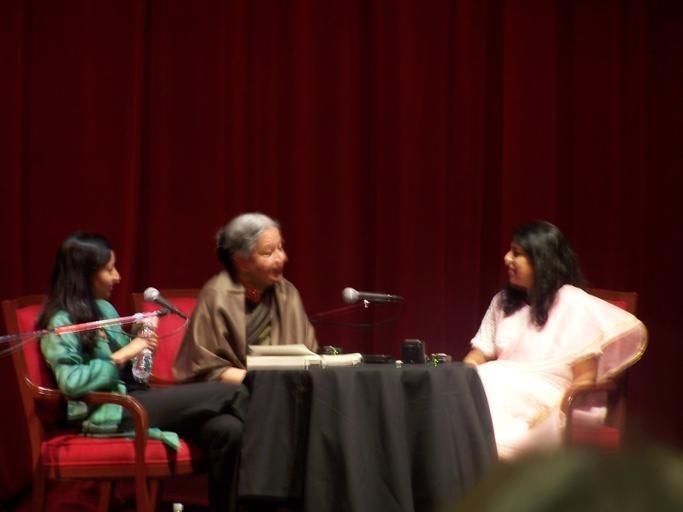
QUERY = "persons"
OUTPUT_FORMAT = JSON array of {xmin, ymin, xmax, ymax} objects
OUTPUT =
[
  {"xmin": 35, "ymin": 235, "xmax": 252, "ymax": 511},
  {"xmin": 170, "ymin": 214, "xmax": 319, "ymax": 383},
  {"xmin": 464, "ymin": 222, "xmax": 647, "ymax": 465}
]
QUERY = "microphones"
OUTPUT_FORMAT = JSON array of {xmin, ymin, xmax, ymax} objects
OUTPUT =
[
  {"xmin": 143, "ymin": 287, "xmax": 188, "ymax": 320},
  {"xmin": 341, "ymin": 286, "xmax": 400, "ymax": 305}
]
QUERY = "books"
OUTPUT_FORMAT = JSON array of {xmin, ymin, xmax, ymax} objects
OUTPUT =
[
  {"xmin": 247, "ymin": 338, "xmax": 323, "ymax": 370},
  {"xmin": 320, "ymin": 352, "xmax": 362, "ymax": 367}
]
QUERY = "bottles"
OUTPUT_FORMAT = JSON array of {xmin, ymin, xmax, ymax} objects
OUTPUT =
[{"xmin": 132, "ymin": 318, "xmax": 155, "ymax": 383}]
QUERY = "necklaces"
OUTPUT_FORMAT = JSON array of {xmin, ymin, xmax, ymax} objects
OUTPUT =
[{"xmin": 247, "ymin": 288, "xmax": 257, "ymax": 297}]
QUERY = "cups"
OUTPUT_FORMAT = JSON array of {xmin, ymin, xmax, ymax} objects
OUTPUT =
[{"xmin": 400, "ymin": 338, "xmax": 425, "ymax": 364}]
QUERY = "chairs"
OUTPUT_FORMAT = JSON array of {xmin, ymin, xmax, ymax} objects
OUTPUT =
[
  {"xmin": 2, "ymin": 295, "xmax": 207, "ymax": 511},
  {"xmin": 128, "ymin": 287, "xmax": 203, "ymax": 387},
  {"xmin": 559, "ymin": 289, "xmax": 648, "ymax": 452}
]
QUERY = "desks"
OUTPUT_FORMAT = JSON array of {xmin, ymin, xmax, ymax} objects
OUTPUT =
[{"xmin": 237, "ymin": 359, "xmax": 499, "ymax": 511}]
